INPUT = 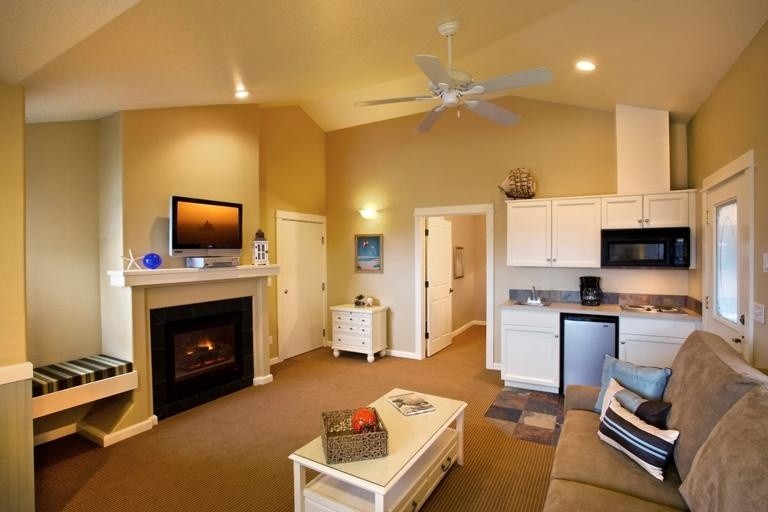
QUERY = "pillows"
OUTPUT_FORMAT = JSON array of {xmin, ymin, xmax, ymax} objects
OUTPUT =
[
  {"xmin": 596, "ymin": 378, "xmax": 673, "ymax": 427},
  {"xmin": 597, "ymin": 397, "xmax": 681, "ymax": 483},
  {"xmin": 592, "ymin": 351, "xmax": 674, "ymax": 413}
]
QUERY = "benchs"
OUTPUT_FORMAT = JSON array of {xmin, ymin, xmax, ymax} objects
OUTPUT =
[{"xmin": 32, "ymin": 354, "xmax": 136, "ymax": 420}]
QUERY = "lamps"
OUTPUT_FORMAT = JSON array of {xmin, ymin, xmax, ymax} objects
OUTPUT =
[{"xmin": 355, "ymin": 208, "xmax": 378, "ymax": 219}]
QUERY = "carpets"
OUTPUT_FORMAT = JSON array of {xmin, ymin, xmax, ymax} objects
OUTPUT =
[{"xmin": 483, "ymin": 386, "xmax": 564, "ymax": 447}]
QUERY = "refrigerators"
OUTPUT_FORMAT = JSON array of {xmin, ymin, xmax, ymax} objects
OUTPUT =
[{"xmin": 560, "ymin": 315, "xmax": 618, "ymax": 398}]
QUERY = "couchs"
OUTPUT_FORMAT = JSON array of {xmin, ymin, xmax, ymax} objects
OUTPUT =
[{"xmin": 544, "ymin": 332, "xmax": 768, "ymax": 512}]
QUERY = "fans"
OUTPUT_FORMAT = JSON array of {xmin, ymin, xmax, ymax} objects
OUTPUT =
[{"xmin": 354, "ymin": 21, "xmax": 559, "ymax": 134}]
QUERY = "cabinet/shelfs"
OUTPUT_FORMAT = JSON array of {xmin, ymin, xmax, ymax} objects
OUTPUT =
[
  {"xmin": 501, "ymin": 312, "xmax": 562, "ymax": 396},
  {"xmin": 505, "ymin": 196, "xmax": 600, "ymax": 268},
  {"xmin": 601, "ymin": 189, "xmax": 702, "ymax": 228},
  {"xmin": 329, "ymin": 305, "xmax": 387, "ymax": 363},
  {"xmin": 618, "ymin": 318, "xmax": 695, "ymax": 379}
]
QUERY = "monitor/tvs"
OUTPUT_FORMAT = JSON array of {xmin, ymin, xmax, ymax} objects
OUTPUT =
[{"xmin": 168, "ymin": 194, "xmax": 243, "ymax": 257}]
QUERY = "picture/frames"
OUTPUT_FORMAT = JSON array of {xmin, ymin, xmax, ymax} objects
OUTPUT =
[
  {"xmin": 452, "ymin": 246, "xmax": 465, "ymax": 280},
  {"xmin": 354, "ymin": 233, "xmax": 383, "ymax": 274}
]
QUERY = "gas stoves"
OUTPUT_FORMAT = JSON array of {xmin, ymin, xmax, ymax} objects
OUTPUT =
[{"xmin": 618, "ymin": 302, "xmax": 689, "ymax": 316}]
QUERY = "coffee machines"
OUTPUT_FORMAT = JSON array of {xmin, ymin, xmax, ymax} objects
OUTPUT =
[{"xmin": 578, "ymin": 275, "xmax": 602, "ymax": 306}]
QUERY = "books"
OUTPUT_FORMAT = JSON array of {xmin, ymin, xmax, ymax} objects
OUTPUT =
[{"xmin": 386, "ymin": 393, "xmax": 436, "ymax": 416}]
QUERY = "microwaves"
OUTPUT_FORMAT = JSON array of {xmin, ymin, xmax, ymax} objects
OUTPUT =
[{"xmin": 599, "ymin": 226, "xmax": 690, "ymax": 268}]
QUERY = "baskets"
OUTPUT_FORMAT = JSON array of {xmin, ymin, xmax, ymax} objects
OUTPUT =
[{"xmin": 320, "ymin": 407, "xmax": 388, "ymax": 463}]
